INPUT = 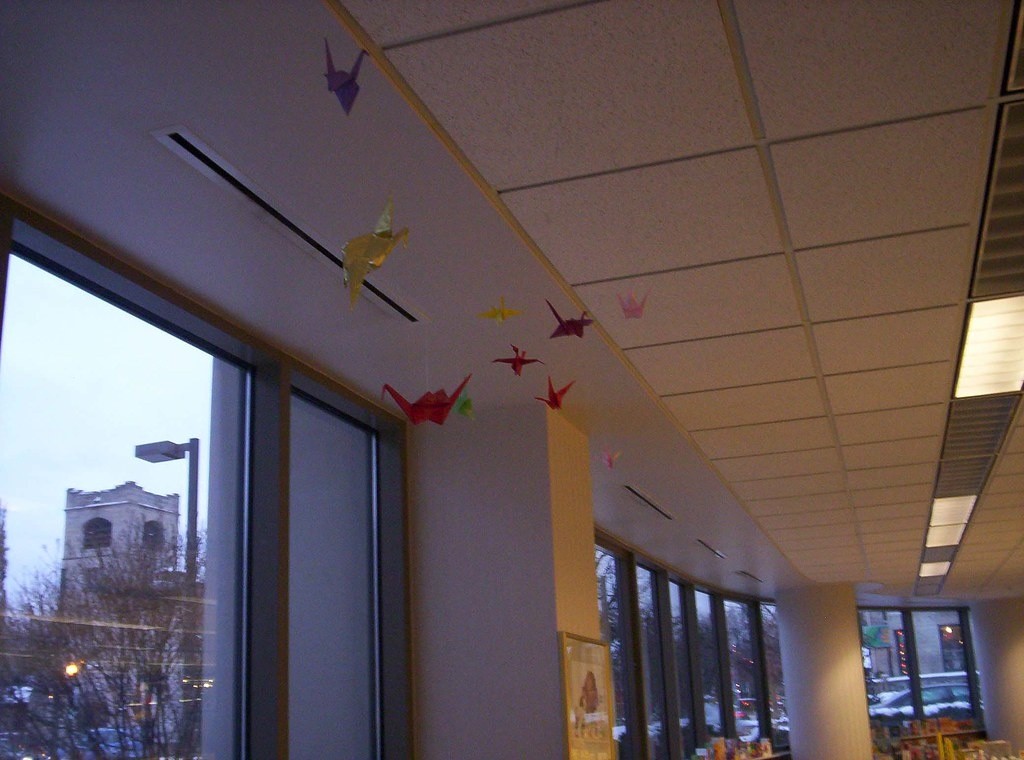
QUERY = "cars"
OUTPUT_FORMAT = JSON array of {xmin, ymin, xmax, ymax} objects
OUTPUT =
[
  {"xmin": 868, "ymin": 680, "xmax": 981, "ymax": 712},
  {"xmin": 611, "ymin": 690, "xmax": 790, "ymax": 759}
]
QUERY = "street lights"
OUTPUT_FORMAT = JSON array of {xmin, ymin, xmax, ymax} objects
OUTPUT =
[{"xmin": 134, "ymin": 435, "xmax": 201, "ymax": 760}]
